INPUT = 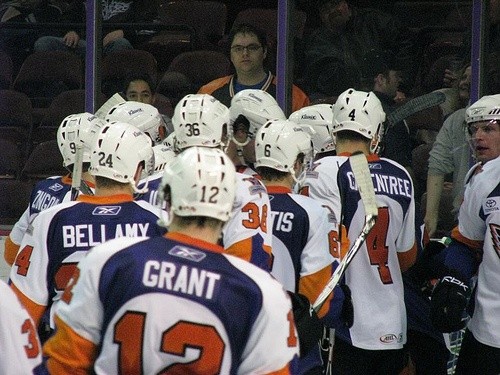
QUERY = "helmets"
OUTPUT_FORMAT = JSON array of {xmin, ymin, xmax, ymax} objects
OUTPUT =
[
  {"xmin": 86, "ymin": 120, "xmax": 156, "ymax": 184},
  {"xmin": 104, "ymin": 100, "xmax": 171, "ymax": 142},
  {"xmin": 464, "ymin": 93, "xmax": 500, "ymax": 124},
  {"xmin": 160, "ymin": 146, "xmax": 236, "ymax": 222},
  {"xmin": 56, "ymin": 111, "xmax": 108, "ymax": 168},
  {"xmin": 251, "ymin": 118, "xmax": 315, "ymax": 173},
  {"xmin": 329, "ymin": 88, "xmax": 386, "ymax": 139},
  {"xmin": 171, "ymin": 92, "xmax": 232, "ymax": 152},
  {"xmin": 287, "ymin": 103, "xmax": 337, "ymax": 158},
  {"xmin": 229, "ymin": 89, "xmax": 287, "ymax": 138}
]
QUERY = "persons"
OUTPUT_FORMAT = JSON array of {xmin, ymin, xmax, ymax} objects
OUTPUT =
[
  {"xmin": 0, "ymin": 0, "xmax": 500, "ymax": 375},
  {"xmin": 197, "ymin": 23, "xmax": 310, "ymax": 119}
]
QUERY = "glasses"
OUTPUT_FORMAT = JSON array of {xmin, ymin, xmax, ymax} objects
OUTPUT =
[{"xmin": 229, "ymin": 44, "xmax": 263, "ymax": 54}]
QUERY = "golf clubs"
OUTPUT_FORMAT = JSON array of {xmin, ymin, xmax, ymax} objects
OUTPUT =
[
  {"xmin": 311, "ymin": 151, "xmax": 380, "ymax": 314},
  {"xmin": 385, "ymin": 91, "xmax": 448, "ymax": 139}
]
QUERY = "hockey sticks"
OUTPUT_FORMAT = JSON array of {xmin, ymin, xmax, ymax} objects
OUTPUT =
[{"xmin": 70, "ymin": 142, "xmax": 85, "ymax": 200}]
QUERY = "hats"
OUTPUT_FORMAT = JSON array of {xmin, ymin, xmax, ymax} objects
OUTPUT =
[{"xmin": 356, "ymin": 48, "xmax": 413, "ymax": 78}]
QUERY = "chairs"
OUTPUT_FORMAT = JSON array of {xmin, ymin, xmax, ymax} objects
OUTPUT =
[{"xmin": 0, "ymin": 0, "xmax": 500, "ymax": 224}]
